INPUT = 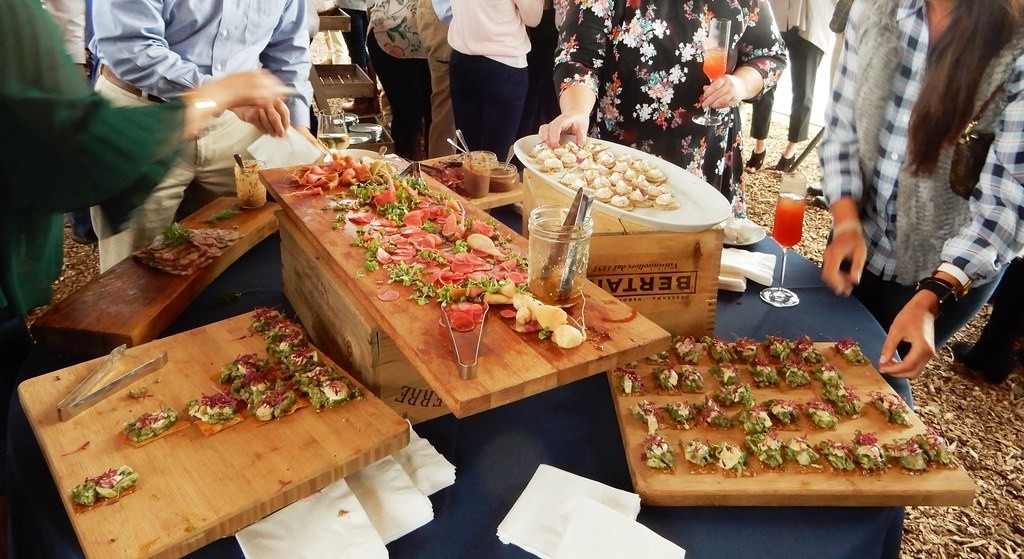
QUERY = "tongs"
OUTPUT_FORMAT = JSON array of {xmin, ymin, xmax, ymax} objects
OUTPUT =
[
  {"xmin": 439, "ymin": 298, "xmax": 490, "ymax": 380},
  {"xmin": 56, "ymin": 344, "xmax": 169, "ymax": 423},
  {"xmin": 398, "ymin": 161, "xmax": 422, "ymax": 185}
]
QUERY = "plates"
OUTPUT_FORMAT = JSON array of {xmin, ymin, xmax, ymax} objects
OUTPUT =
[
  {"xmin": 723, "ymin": 220, "xmax": 765, "ymax": 245},
  {"xmin": 514, "ymin": 135, "xmax": 732, "ymax": 234},
  {"xmin": 333, "ymin": 111, "xmax": 382, "ymax": 145},
  {"xmin": 323, "ymin": 149, "xmax": 380, "ymax": 163}
]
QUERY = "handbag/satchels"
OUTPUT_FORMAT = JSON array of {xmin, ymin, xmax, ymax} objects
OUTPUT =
[{"xmin": 949, "ymin": 79, "xmax": 1011, "ymax": 201}]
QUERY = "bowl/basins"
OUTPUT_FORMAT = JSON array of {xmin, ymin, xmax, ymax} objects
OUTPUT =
[
  {"xmin": 489, "ymin": 161, "xmax": 520, "ymax": 192},
  {"xmin": 469, "ymin": 150, "xmax": 499, "ymax": 165}
]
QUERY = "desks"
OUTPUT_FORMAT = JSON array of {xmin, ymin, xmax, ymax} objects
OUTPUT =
[{"xmin": 7, "ymin": 205, "xmax": 914, "ymax": 559}]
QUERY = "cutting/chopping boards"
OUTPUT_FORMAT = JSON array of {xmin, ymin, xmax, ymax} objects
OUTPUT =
[
  {"xmin": 16, "ymin": 309, "xmax": 411, "ymax": 559},
  {"xmin": 415, "ymin": 154, "xmax": 523, "ymax": 210},
  {"xmin": 258, "ymin": 155, "xmax": 672, "ymax": 419},
  {"xmin": 29, "ymin": 196, "xmax": 281, "ymax": 350},
  {"xmin": 607, "ymin": 341, "xmax": 976, "ymax": 507}
]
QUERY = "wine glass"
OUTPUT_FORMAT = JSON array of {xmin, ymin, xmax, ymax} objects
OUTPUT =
[
  {"xmin": 692, "ymin": 17, "xmax": 731, "ymax": 126},
  {"xmin": 760, "ymin": 173, "xmax": 807, "ymax": 307}
]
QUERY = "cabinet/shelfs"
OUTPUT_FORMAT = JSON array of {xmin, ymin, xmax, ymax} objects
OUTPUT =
[{"xmin": 308, "ymin": 8, "xmax": 395, "ymax": 154}]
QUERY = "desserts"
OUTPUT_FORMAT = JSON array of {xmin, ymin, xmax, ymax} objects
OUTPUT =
[{"xmin": 526, "ymin": 139, "xmax": 679, "ymax": 212}]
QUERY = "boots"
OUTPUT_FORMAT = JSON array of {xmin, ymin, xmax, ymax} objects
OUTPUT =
[{"xmin": 339, "ymin": 61, "xmax": 380, "ymax": 115}]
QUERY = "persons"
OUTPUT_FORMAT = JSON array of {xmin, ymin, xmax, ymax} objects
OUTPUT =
[
  {"xmin": 816, "ymin": 0, "xmax": 1024, "ymax": 385},
  {"xmin": 363, "ymin": 0, "xmax": 432, "ymax": 161},
  {"xmin": 42, "ymin": 0, "xmax": 97, "ymax": 243},
  {"xmin": 515, "ymin": 1, "xmax": 558, "ymax": 144},
  {"xmin": 743, "ymin": 0, "xmax": 831, "ymax": 173},
  {"xmin": 445, "ymin": 1, "xmax": 547, "ymax": 161},
  {"xmin": 86, "ymin": 1, "xmax": 330, "ymax": 275},
  {"xmin": 415, "ymin": 1, "xmax": 458, "ymax": 159},
  {"xmin": 335, "ymin": 0, "xmax": 380, "ymax": 113},
  {"xmin": 537, "ymin": 0, "xmax": 790, "ymax": 215},
  {"xmin": 0, "ymin": 0, "xmax": 295, "ymax": 502}
]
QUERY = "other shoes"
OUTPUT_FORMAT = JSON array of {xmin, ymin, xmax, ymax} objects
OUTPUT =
[
  {"xmin": 952, "ymin": 341, "xmax": 1000, "ymax": 384},
  {"xmin": 807, "ymin": 182, "xmax": 824, "ymax": 196},
  {"xmin": 746, "ymin": 146, "xmax": 766, "ymax": 173},
  {"xmin": 776, "ymin": 154, "xmax": 796, "ymax": 171},
  {"xmin": 814, "ymin": 195, "xmax": 828, "ymax": 209}
]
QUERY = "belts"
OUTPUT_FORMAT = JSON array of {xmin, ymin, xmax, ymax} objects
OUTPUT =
[{"xmin": 100, "ymin": 65, "xmax": 165, "ymax": 103}]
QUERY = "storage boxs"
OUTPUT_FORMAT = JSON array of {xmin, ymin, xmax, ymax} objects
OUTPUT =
[
  {"xmin": 273, "ymin": 208, "xmax": 454, "ymax": 424},
  {"xmin": 522, "ymin": 169, "xmax": 725, "ymax": 343}
]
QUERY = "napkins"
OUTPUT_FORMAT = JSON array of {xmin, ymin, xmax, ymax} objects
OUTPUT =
[
  {"xmin": 718, "ymin": 216, "xmax": 777, "ymax": 294},
  {"xmin": 235, "ymin": 418, "xmax": 457, "ymax": 559},
  {"xmin": 496, "ymin": 462, "xmax": 687, "ymax": 559}
]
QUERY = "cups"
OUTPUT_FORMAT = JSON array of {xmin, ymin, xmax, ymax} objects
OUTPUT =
[
  {"xmin": 464, "ymin": 154, "xmax": 490, "ymax": 198},
  {"xmin": 233, "ymin": 159, "xmax": 267, "ymax": 209},
  {"xmin": 317, "ymin": 108, "xmax": 350, "ymax": 155}
]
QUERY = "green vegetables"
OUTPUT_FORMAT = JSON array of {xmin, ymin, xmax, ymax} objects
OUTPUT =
[
  {"xmin": 321, "ymin": 176, "xmax": 550, "ymax": 340},
  {"xmin": 162, "ymin": 205, "xmax": 242, "ymax": 246}
]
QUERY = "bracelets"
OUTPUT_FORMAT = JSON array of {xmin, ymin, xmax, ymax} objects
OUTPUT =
[
  {"xmin": 833, "ymin": 220, "xmax": 865, "ymax": 240},
  {"xmin": 930, "ymin": 261, "xmax": 973, "ymax": 295}
]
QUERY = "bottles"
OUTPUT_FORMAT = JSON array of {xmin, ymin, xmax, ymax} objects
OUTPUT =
[{"xmin": 527, "ymin": 205, "xmax": 593, "ymax": 308}]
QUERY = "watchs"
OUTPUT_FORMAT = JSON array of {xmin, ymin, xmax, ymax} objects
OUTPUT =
[{"xmin": 913, "ymin": 276, "xmax": 958, "ymax": 319}]
